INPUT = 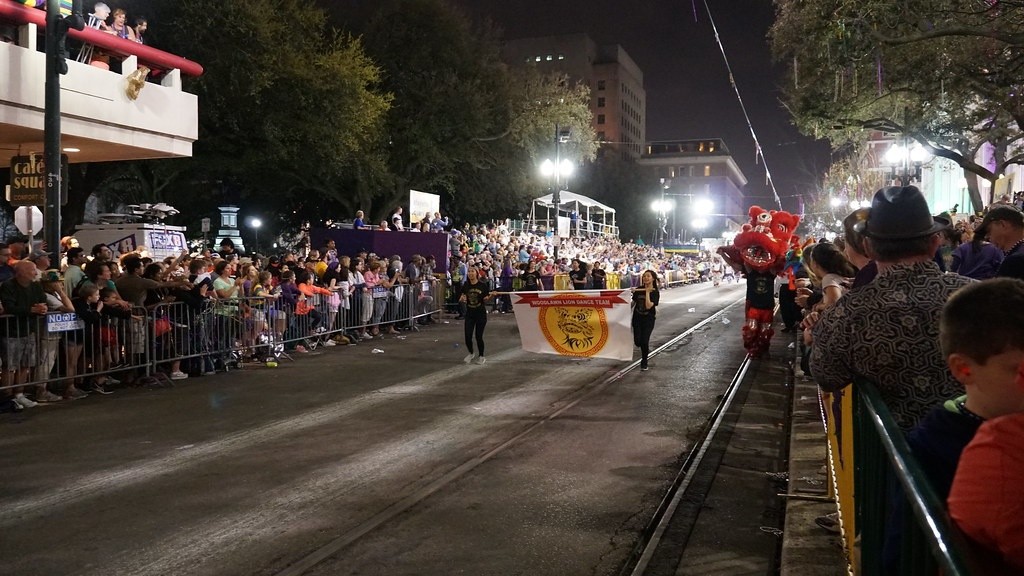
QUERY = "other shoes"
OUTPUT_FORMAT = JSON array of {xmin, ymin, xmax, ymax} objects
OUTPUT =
[
  {"xmin": 387, "ymin": 330, "xmax": 400, "ymax": 334},
  {"xmin": 370, "ymin": 331, "xmax": 383, "ymax": 338},
  {"xmin": 36, "ymin": 390, "xmax": 63, "ymax": 402},
  {"xmin": 91, "ymin": 380, "xmax": 116, "ymax": 394},
  {"xmin": 170, "ymin": 369, "xmax": 188, "ymax": 380},
  {"xmin": 63, "ymin": 388, "xmax": 89, "ymax": 400},
  {"xmin": 204, "ymin": 368, "xmax": 212, "ymax": 375},
  {"xmin": 639, "ymin": 359, "xmax": 649, "ymax": 370},
  {"xmin": 297, "ymin": 345, "xmax": 309, "ymax": 353},
  {"xmin": 360, "ymin": 332, "xmax": 374, "ymax": 340},
  {"xmin": 107, "ymin": 376, "xmax": 119, "ymax": 384},
  {"xmin": 781, "ymin": 325, "xmax": 796, "ymax": 331},
  {"xmin": 816, "ymin": 512, "xmax": 842, "ymax": 533},
  {"xmin": 323, "ymin": 339, "xmax": 339, "ymax": 346}
]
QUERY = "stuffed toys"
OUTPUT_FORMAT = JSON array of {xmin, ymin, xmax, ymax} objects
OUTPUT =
[{"xmin": 720, "ymin": 206, "xmax": 799, "ymax": 268}]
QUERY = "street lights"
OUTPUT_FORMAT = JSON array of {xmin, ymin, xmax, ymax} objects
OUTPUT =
[
  {"xmin": 651, "ymin": 198, "xmax": 672, "ymax": 242},
  {"xmin": 883, "ymin": 105, "xmax": 924, "ymax": 186},
  {"xmin": 537, "ymin": 157, "xmax": 574, "ymax": 260}
]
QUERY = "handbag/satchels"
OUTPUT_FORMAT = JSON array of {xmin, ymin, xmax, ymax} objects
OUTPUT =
[{"xmin": 155, "ymin": 315, "xmax": 172, "ymax": 337}]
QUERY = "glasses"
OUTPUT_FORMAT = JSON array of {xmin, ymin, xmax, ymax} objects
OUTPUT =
[{"xmin": 78, "ymin": 254, "xmax": 86, "ymax": 258}]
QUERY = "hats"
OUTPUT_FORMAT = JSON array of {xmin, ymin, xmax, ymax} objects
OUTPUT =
[
  {"xmin": 41, "ymin": 269, "xmax": 65, "ymax": 281},
  {"xmin": 973, "ymin": 205, "xmax": 1022, "ymax": 236},
  {"xmin": 31, "ymin": 249, "xmax": 53, "ymax": 261},
  {"xmin": 319, "ymin": 247, "xmax": 330, "ymax": 259},
  {"xmin": 468, "ymin": 264, "xmax": 479, "ymax": 279},
  {"xmin": 857, "ymin": 185, "xmax": 952, "ymax": 239}
]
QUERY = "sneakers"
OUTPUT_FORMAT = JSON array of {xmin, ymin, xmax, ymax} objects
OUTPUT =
[
  {"xmin": 464, "ymin": 353, "xmax": 476, "ymax": 363},
  {"xmin": 16, "ymin": 395, "xmax": 39, "ymax": 408},
  {"xmin": 5, "ymin": 396, "xmax": 24, "ymax": 410},
  {"xmin": 475, "ymin": 356, "xmax": 486, "ymax": 364}
]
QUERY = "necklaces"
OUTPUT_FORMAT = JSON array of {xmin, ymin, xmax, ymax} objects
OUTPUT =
[{"xmin": 1007, "ymin": 238, "xmax": 1024, "ymax": 254}]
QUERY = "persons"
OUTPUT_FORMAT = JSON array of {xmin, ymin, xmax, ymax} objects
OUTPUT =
[
  {"xmin": 390, "ymin": 218, "xmax": 401, "ymax": 232},
  {"xmin": 774, "ymin": 186, "xmax": 1024, "ymax": 576},
  {"xmin": 354, "ymin": 211, "xmax": 369, "ymax": 229},
  {"xmin": 458, "ymin": 265, "xmax": 497, "ymax": 364},
  {"xmin": 579, "ymin": 214, "xmax": 585, "ymax": 228},
  {"xmin": 0, "ymin": 237, "xmax": 435, "ymax": 412},
  {"xmin": 631, "ymin": 270, "xmax": 659, "ymax": 371},
  {"xmin": 392, "ymin": 207, "xmax": 404, "ymax": 231},
  {"xmin": 374, "ymin": 221, "xmax": 391, "ymax": 231},
  {"xmin": 420, "ymin": 212, "xmax": 448, "ymax": 232},
  {"xmin": 24, "ymin": 0, "xmax": 149, "ymax": 80},
  {"xmin": 446, "ymin": 220, "xmax": 723, "ymax": 314},
  {"xmin": 410, "ymin": 222, "xmax": 421, "ymax": 232},
  {"xmin": 570, "ymin": 210, "xmax": 576, "ymax": 228},
  {"xmin": 717, "ymin": 247, "xmax": 785, "ymax": 359}
]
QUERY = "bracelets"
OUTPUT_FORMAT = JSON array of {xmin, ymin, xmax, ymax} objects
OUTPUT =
[{"xmin": 814, "ymin": 305, "xmax": 817, "ymax": 310}]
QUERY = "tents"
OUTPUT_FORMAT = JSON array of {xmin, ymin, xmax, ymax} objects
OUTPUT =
[{"xmin": 532, "ymin": 190, "xmax": 616, "ymax": 235}]
